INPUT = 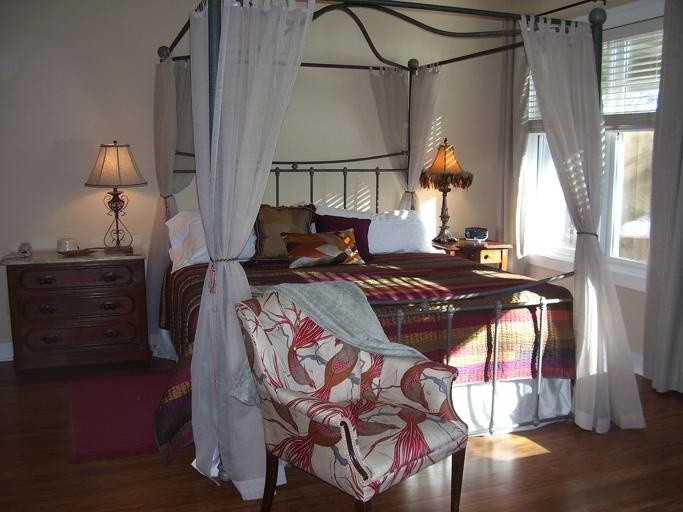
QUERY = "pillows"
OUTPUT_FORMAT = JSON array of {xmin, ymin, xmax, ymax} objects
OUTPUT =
[
  {"xmin": 248, "ymin": 201, "xmax": 319, "ymax": 267},
  {"xmin": 307, "ymin": 194, "xmax": 445, "ymax": 256},
  {"xmin": 158, "ymin": 206, "xmax": 256, "ymax": 275},
  {"xmin": 277, "ymin": 224, "xmax": 370, "ymax": 273},
  {"xmin": 314, "ymin": 210, "xmax": 374, "ymax": 260}
]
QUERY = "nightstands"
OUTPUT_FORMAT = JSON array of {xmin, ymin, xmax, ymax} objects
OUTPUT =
[
  {"xmin": 434, "ymin": 237, "xmax": 515, "ymax": 271},
  {"xmin": 0, "ymin": 243, "xmax": 151, "ymax": 387}
]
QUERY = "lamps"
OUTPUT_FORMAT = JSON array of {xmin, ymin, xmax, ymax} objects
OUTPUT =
[
  {"xmin": 81, "ymin": 139, "xmax": 149, "ymax": 257},
  {"xmin": 416, "ymin": 133, "xmax": 476, "ymax": 245}
]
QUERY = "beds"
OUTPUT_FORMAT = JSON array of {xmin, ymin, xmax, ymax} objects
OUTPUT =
[{"xmin": 137, "ymin": 0, "xmax": 652, "ymax": 505}]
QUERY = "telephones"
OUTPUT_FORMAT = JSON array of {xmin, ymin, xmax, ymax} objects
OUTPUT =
[{"xmin": 16, "ymin": 243, "xmax": 33, "ymax": 258}]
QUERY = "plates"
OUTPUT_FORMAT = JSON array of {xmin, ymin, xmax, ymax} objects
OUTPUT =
[{"xmin": 58, "ymin": 250, "xmax": 95, "ymax": 256}]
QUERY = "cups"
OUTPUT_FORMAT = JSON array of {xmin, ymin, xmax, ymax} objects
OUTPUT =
[{"xmin": 56, "ymin": 238, "xmax": 77, "ymax": 251}]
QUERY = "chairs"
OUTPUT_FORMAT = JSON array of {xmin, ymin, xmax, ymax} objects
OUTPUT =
[{"xmin": 231, "ymin": 289, "xmax": 475, "ymax": 511}]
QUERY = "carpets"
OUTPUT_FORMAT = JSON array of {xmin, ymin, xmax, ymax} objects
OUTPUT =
[{"xmin": 58, "ymin": 362, "xmax": 186, "ymax": 470}]
